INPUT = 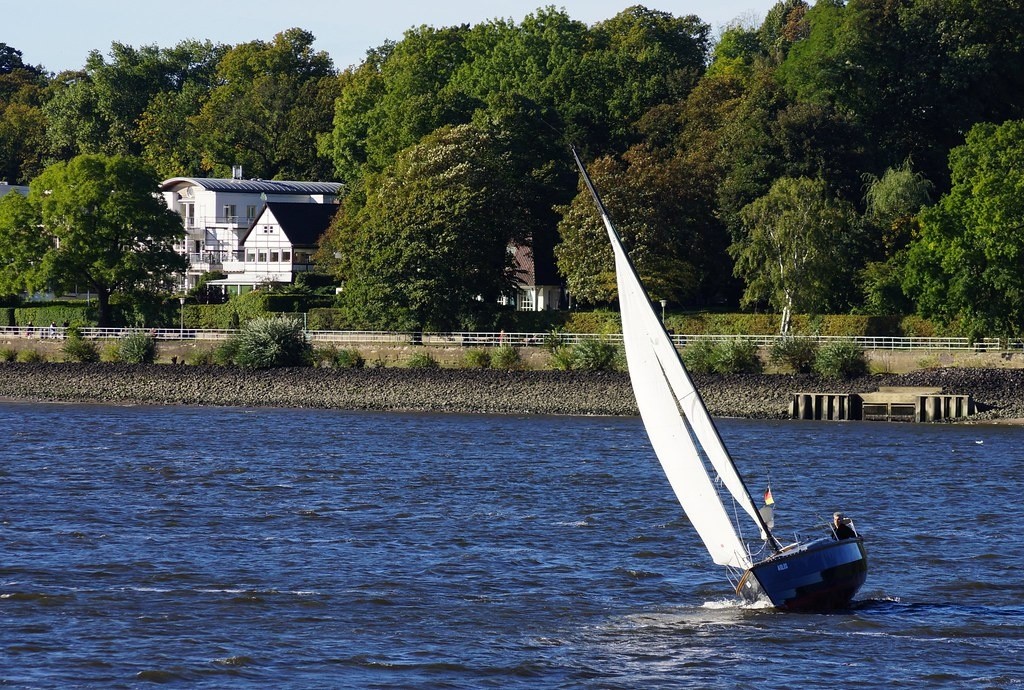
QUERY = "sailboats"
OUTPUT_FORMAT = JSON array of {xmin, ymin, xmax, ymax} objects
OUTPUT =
[{"xmin": 571, "ymin": 148, "xmax": 867, "ymax": 612}]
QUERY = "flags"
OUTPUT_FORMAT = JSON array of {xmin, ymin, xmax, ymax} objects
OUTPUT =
[{"xmin": 764, "ymin": 486, "xmax": 774, "ymax": 506}]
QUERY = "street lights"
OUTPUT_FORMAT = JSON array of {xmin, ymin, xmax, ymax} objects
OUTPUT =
[
  {"xmin": 179, "ymin": 297, "xmax": 186, "ymax": 341},
  {"xmin": 658, "ymin": 300, "xmax": 667, "ymax": 325}
]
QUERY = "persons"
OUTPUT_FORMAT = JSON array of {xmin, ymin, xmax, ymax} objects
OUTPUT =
[
  {"xmin": 61, "ymin": 319, "xmax": 70, "ymax": 340},
  {"xmin": 50, "ymin": 321, "xmax": 58, "ymax": 339},
  {"xmin": 499, "ymin": 330, "xmax": 508, "ymax": 346},
  {"xmin": 831, "ymin": 512, "xmax": 863, "ymax": 541},
  {"xmin": 25, "ymin": 321, "xmax": 34, "ymax": 337}
]
